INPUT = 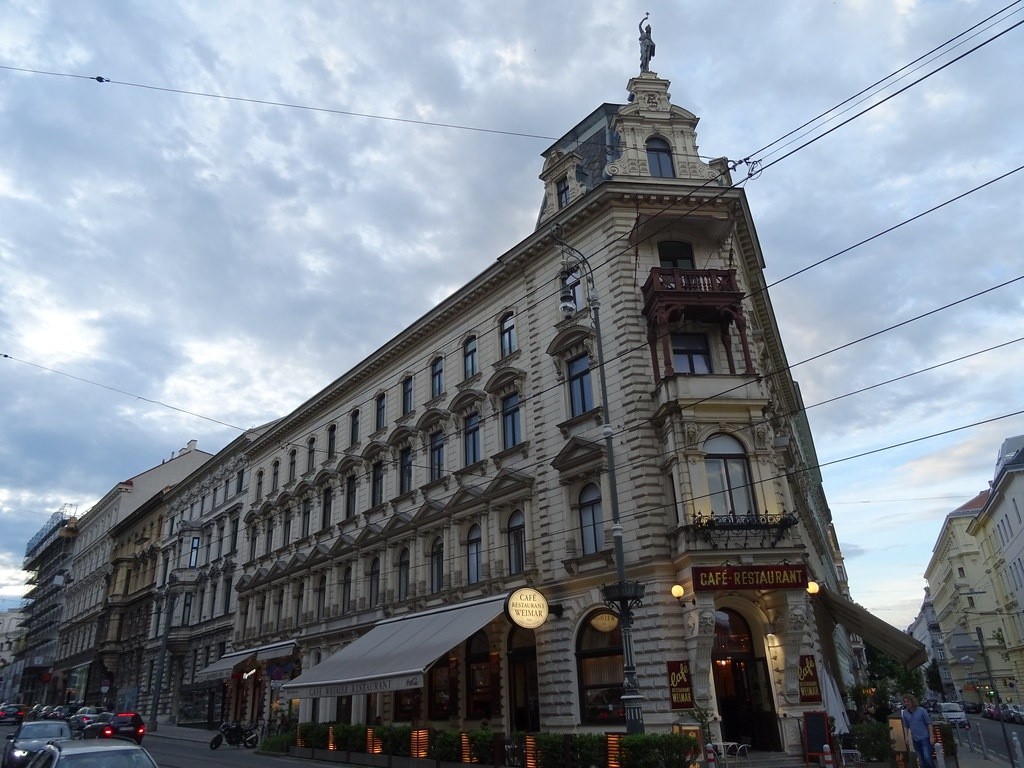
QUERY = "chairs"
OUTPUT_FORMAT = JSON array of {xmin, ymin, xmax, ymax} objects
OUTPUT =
[
  {"xmin": 840, "ymin": 736, "xmax": 860, "ymax": 766},
  {"xmin": 728, "ymin": 737, "xmax": 752, "ymax": 768}
]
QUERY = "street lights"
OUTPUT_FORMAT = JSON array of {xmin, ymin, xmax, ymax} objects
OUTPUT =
[
  {"xmin": 556, "ymin": 237, "xmax": 644, "ymax": 735},
  {"xmin": 953, "ymin": 631, "xmax": 1015, "ymax": 768}
]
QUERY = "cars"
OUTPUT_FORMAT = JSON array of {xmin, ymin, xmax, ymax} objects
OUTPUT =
[
  {"xmin": 981, "ymin": 702, "xmax": 1024, "ymax": 724},
  {"xmin": 25, "ymin": 733, "xmax": 159, "ymax": 768},
  {"xmin": 963, "ymin": 702, "xmax": 980, "ymax": 714},
  {"xmin": 894, "ymin": 703, "xmax": 904, "ymax": 710},
  {"xmin": 2, "ymin": 721, "xmax": 82, "ymax": 768},
  {"xmin": 938, "ymin": 702, "xmax": 972, "ymax": 728},
  {"xmin": 29, "ymin": 704, "xmax": 81, "ymax": 721}
]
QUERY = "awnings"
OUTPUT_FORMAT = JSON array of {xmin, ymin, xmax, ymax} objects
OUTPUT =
[
  {"xmin": 819, "ymin": 586, "xmax": 928, "ymax": 672},
  {"xmin": 197, "ymin": 652, "xmax": 255, "ymax": 683},
  {"xmin": 282, "ymin": 599, "xmax": 504, "ymax": 700}
]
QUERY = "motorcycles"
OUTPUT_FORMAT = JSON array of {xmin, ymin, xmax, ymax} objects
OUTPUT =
[{"xmin": 209, "ymin": 713, "xmax": 260, "ymax": 751}]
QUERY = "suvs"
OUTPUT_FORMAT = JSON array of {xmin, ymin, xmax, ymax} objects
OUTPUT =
[
  {"xmin": 0, "ymin": 703, "xmax": 26, "ymax": 725},
  {"xmin": 68, "ymin": 705, "xmax": 109, "ymax": 730},
  {"xmin": 79, "ymin": 711, "xmax": 146, "ymax": 745}
]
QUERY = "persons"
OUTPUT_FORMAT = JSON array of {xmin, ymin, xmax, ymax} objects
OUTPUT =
[
  {"xmin": 873, "ymin": 700, "xmax": 892, "ymax": 724},
  {"xmin": 638, "ymin": 17, "xmax": 656, "ymax": 71},
  {"xmin": 984, "ymin": 701, "xmax": 988, "ymax": 705},
  {"xmin": 901, "ymin": 694, "xmax": 937, "ymax": 768},
  {"xmin": 926, "ymin": 700, "xmax": 934, "ymax": 713}
]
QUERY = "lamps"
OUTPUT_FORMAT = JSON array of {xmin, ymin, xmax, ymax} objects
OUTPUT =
[
  {"xmin": 672, "ymin": 585, "xmax": 696, "ymax": 606},
  {"xmin": 806, "ymin": 583, "xmax": 821, "ymax": 603}
]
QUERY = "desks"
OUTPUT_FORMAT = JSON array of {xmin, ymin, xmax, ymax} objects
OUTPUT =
[{"xmin": 712, "ymin": 742, "xmax": 737, "ymax": 768}]
